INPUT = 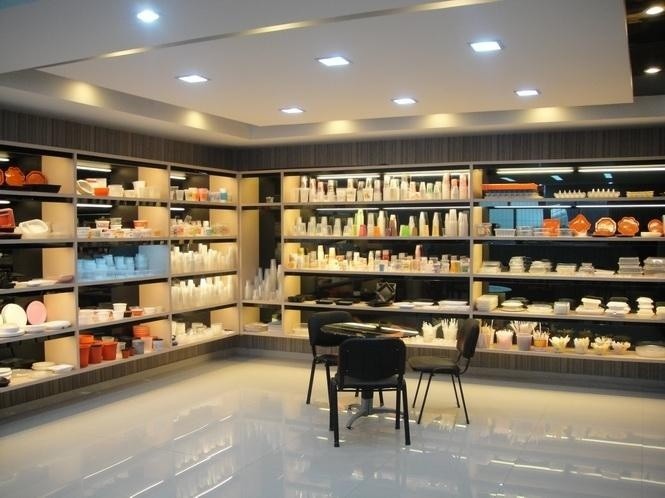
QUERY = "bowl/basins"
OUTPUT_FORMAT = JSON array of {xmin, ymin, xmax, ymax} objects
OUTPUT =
[
  {"xmin": 78, "ymin": 325, "xmax": 167, "ymax": 369},
  {"xmin": 0, "ymin": 367, "xmax": 12, "ymax": 387},
  {"xmin": 23, "ymin": 184, "xmax": 62, "ymax": 192},
  {"xmin": 94, "ymin": 187, "xmax": 109, "ymax": 196},
  {"xmin": 76, "ymin": 179, "xmax": 94, "ymax": 196}
]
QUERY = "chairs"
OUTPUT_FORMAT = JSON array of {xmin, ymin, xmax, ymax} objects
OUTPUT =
[
  {"xmin": 306, "ymin": 310, "xmax": 386, "ymax": 408},
  {"xmin": 330, "ymin": 337, "xmax": 410, "ymax": 447},
  {"xmin": 408, "ymin": 318, "xmax": 480, "ymax": 425}
]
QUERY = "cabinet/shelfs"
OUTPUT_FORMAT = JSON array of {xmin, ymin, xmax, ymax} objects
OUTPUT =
[
  {"xmin": 238, "ymin": 156, "xmax": 665, "ymax": 364},
  {"xmin": 0, "ymin": 140, "xmax": 240, "ymax": 394}
]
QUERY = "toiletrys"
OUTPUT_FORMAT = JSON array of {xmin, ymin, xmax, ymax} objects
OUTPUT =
[
  {"xmin": 76, "ymin": 186, "xmax": 282, "ymax": 312},
  {"xmin": 287, "ymin": 172, "xmax": 470, "ymax": 275}
]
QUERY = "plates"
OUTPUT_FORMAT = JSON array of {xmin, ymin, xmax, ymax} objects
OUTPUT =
[
  {"xmin": 245, "ymin": 322, "xmax": 268, "ymax": 332},
  {"xmin": 567, "ymin": 213, "xmax": 664, "ymax": 236},
  {"xmin": 542, "ymin": 218, "xmax": 560, "ymax": 236},
  {"xmin": 636, "ymin": 345, "xmax": 665, "ymax": 357},
  {"xmin": 0, "ymin": 300, "xmax": 70, "ymax": 334},
  {"xmin": 395, "ymin": 300, "xmax": 469, "ymax": 311},
  {"xmin": 32, "ymin": 361, "xmax": 73, "ymax": 374},
  {"xmin": 478, "ymin": 294, "xmax": 631, "ymax": 317},
  {"xmin": 365, "ymin": 282, "xmax": 397, "ymax": 308},
  {"xmin": 0, "ymin": 167, "xmax": 47, "ymax": 186}
]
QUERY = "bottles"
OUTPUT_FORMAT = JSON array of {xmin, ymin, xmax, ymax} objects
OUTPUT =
[
  {"xmin": 289, "ymin": 244, "xmax": 468, "ymax": 275},
  {"xmin": 170, "ymin": 277, "xmax": 238, "ymax": 307},
  {"xmin": 293, "ymin": 209, "xmax": 468, "ymax": 237},
  {"xmin": 244, "ymin": 259, "xmax": 283, "ymax": 303},
  {"xmin": 384, "ymin": 173, "xmax": 468, "ymax": 200},
  {"xmin": 172, "ymin": 244, "xmax": 234, "ymax": 273},
  {"xmin": 292, "ymin": 174, "xmax": 381, "ymax": 204},
  {"xmin": 170, "ymin": 215, "xmax": 212, "ymax": 236}
]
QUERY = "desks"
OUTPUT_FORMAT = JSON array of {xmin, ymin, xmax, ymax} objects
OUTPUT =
[{"xmin": 321, "ymin": 321, "xmax": 420, "ymax": 429}]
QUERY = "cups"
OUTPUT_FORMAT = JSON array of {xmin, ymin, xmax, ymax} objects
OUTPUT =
[
  {"xmin": 78, "ymin": 218, "xmax": 160, "ymax": 238},
  {"xmin": 170, "ymin": 185, "xmax": 227, "ymax": 203},
  {"xmin": 108, "ymin": 181, "xmax": 146, "ymax": 198},
  {"xmin": 78, "ymin": 302, "xmax": 156, "ymax": 327},
  {"xmin": 77, "ymin": 253, "xmax": 149, "ymax": 280}
]
